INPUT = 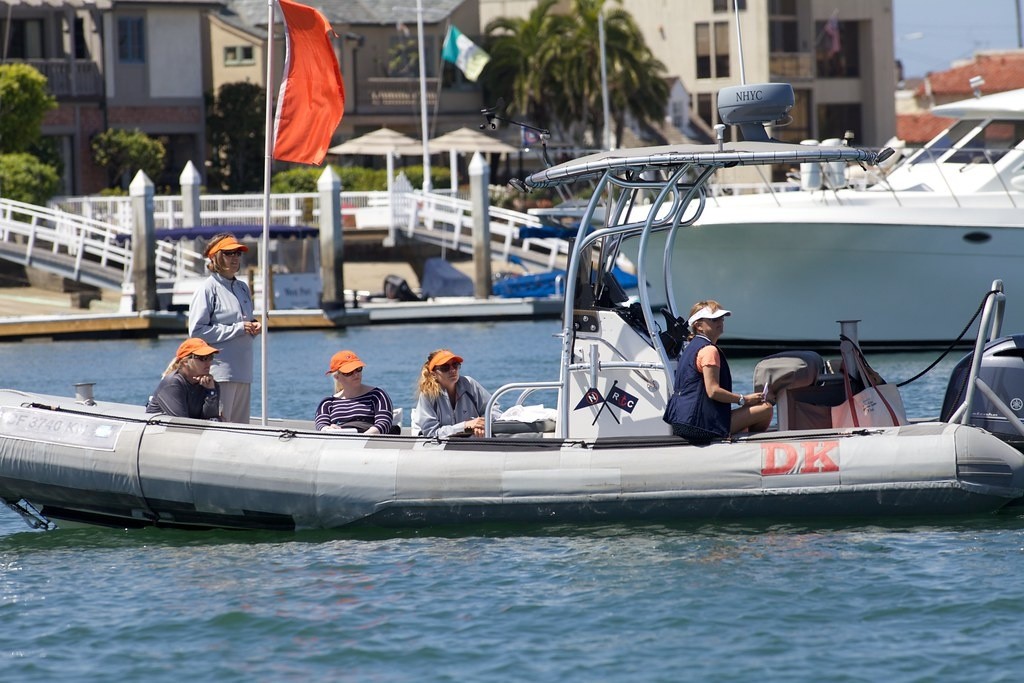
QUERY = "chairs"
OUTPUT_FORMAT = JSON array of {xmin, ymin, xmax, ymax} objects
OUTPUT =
[{"xmin": 776, "ymin": 316, "xmax": 863, "ymax": 432}]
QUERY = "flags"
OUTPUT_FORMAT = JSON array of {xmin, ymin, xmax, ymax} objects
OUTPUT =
[
  {"xmin": 523, "ymin": 126, "xmax": 540, "ymax": 146},
  {"xmin": 439, "ymin": 21, "xmax": 490, "ymax": 84},
  {"xmin": 272, "ymin": 0, "xmax": 346, "ymax": 165},
  {"xmin": 825, "ymin": 9, "xmax": 842, "ymax": 56}
]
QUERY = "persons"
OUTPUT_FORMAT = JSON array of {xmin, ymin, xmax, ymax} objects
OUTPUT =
[
  {"xmin": 415, "ymin": 350, "xmax": 502, "ymax": 440},
  {"xmin": 314, "ymin": 350, "xmax": 393, "ymax": 436},
  {"xmin": 146, "ymin": 335, "xmax": 221, "ymax": 425},
  {"xmin": 187, "ymin": 234, "xmax": 261, "ymax": 425},
  {"xmin": 660, "ymin": 301, "xmax": 774, "ymax": 444}
]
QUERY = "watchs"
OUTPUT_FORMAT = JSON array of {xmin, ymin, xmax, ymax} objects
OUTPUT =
[{"xmin": 739, "ymin": 394, "xmax": 745, "ymax": 405}]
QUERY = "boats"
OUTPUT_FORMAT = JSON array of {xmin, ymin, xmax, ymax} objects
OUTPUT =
[
  {"xmin": 0, "ymin": 79, "xmax": 1024, "ymax": 524},
  {"xmin": 344, "ymin": 273, "xmax": 566, "ymax": 321}
]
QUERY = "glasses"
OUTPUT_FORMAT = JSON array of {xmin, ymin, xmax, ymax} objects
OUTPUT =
[
  {"xmin": 339, "ymin": 366, "xmax": 361, "ymax": 376},
  {"xmin": 702, "ymin": 314, "xmax": 724, "ymax": 323},
  {"xmin": 432, "ymin": 360, "xmax": 462, "ymax": 373},
  {"xmin": 220, "ymin": 249, "xmax": 242, "ymax": 258},
  {"xmin": 189, "ymin": 354, "xmax": 213, "ymax": 362}
]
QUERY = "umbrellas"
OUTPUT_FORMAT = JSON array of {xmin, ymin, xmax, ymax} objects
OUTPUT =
[
  {"xmin": 325, "ymin": 126, "xmax": 429, "ymax": 157},
  {"xmin": 430, "ymin": 124, "xmax": 519, "ymax": 160}
]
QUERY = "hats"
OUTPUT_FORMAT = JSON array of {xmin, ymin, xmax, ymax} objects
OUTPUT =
[
  {"xmin": 175, "ymin": 338, "xmax": 219, "ymax": 359},
  {"xmin": 207, "ymin": 236, "xmax": 248, "ymax": 260},
  {"xmin": 329, "ymin": 350, "xmax": 365, "ymax": 373},
  {"xmin": 688, "ymin": 305, "xmax": 732, "ymax": 326},
  {"xmin": 428, "ymin": 350, "xmax": 464, "ymax": 372}
]
